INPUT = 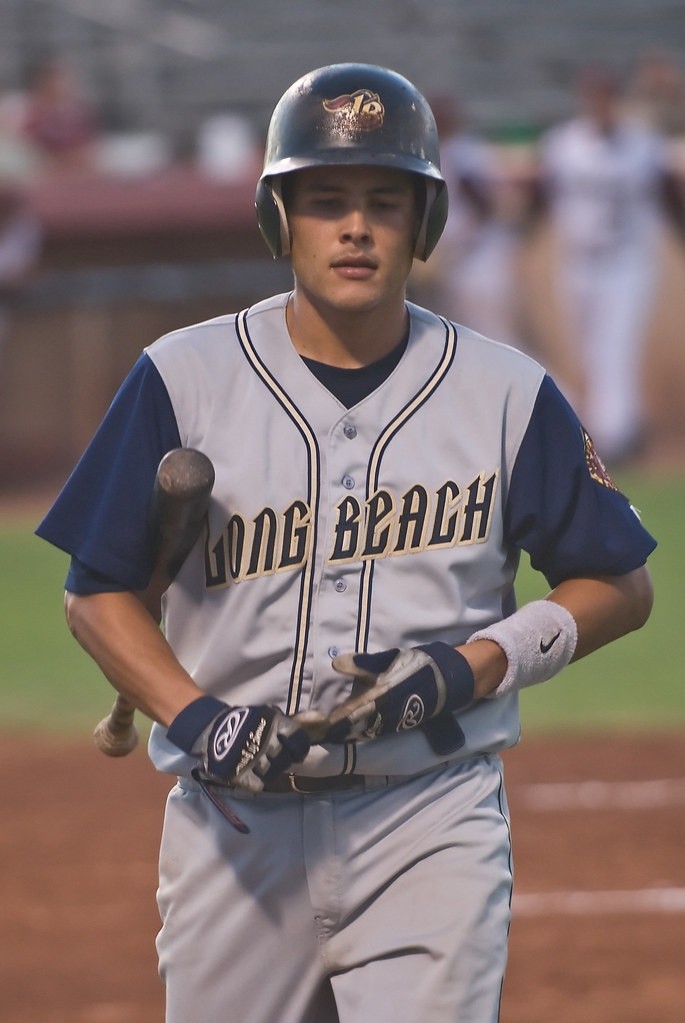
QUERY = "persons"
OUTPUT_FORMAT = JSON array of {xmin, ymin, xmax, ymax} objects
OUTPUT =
[
  {"xmin": 423, "ymin": 92, "xmax": 523, "ymax": 348},
  {"xmin": 34, "ymin": 61, "xmax": 659, "ymax": 1023},
  {"xmin": 523, "ymin": 62, "xmax": 685, "ymax": 464}
]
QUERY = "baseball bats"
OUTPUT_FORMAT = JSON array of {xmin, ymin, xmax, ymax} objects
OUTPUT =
[{"xmin": 93, "ymin": 446, "xmax": 217, "ymax": 758}]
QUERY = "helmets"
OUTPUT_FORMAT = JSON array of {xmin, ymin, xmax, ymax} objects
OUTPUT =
[{"xmin": 254, "ymin": 64, "xmax": 449, "ymax": 264}]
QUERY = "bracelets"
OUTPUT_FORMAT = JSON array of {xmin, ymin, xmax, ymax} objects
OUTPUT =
[{"xmin": 466, "ymin": 599, "xmax": 577, "ymax": 699}]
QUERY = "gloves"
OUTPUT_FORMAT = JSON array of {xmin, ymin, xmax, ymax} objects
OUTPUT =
[
  {"xmin": 166, "ymin": 695, "xmax": 332, "ymax": 834},
  {"xmin": 323, "ymin": 641, "xmax": 475, "ymax": 756}
]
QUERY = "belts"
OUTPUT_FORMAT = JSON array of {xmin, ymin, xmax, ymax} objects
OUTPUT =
[{"xmin": 203, "ymin": 773, "xmax": 364, "ymax": 794}]
